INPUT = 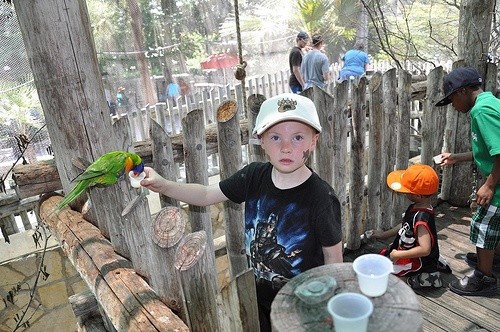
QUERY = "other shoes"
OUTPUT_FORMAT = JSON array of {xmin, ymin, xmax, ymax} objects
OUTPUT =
[
  {"xmin": 437, "ymin": 255, "xmax": 452, "ymax": 273},
  {"xmin": 407, "ymin": 271, "xmax": 443, "ymax": 289}
]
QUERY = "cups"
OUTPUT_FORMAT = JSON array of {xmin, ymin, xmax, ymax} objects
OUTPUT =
[
  {"xmin": 433, "ymin": 155, "xmax": 442, "ymax": 164},
  {"xmin": 129, "ymin": 171, "xmax": 146, "ymax": 187},
  {"xmin": 328, "ymin": 292, "xmax": 373, "ymax": 332},
  {"xmin": 352, "ymin": 253, "xmax": 394, "ymax": 297}
]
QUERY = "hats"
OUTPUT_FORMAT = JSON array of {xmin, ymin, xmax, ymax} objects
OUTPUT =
[
  {"xmin": 252, "ymin": 93, "xmax": 321, "ymax": 139},
  {"xmin": 435, "ymin": 66, "xmax": 482, "ymax": 107},
  {"xmin": 386, "ymin": 164, "xmax": 439, "ymax": 195}
]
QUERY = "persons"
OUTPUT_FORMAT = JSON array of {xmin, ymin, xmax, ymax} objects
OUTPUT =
[
  {"xmin": 116, "ymin": 86, "xmax": 129, "ymax": 105},
  {"xmin": 289, "ymin": 31, "xmax": 311, "ymax": 94},
  {"xmin": 299, "ymin": 33, "xmax": 330, "ymax": 96},
  {"xmin": 369, "ymin": 164, "xmax": 452, "ymax": 291},
  {"xmin": 339, "ymin": 45, "xmax": 370, "ymax": 86},
  {"xmin": 434, "ymin": 67, "xmax": 500, "ymax": 296},
  {"xmin": 140, "ymin": 93, "xmax": 343, "ymax": 332},
  {"xmin": 158, "ymin": 78, "xmax": 189, "ymax": 103}
]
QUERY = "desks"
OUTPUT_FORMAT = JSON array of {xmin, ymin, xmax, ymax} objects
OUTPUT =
[{"xmin": 270, "ymin": 261, "xmax": 423, "ymax": 331}]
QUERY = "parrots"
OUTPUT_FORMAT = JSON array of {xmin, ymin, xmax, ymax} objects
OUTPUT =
[{"xmin": 33, "ymin": 150, "xmax": 145, "ymax": 231}]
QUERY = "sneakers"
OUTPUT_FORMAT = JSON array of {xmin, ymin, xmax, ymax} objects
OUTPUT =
[
  {"xmin": 464, "ymin": 252, "xmax": 500, "ymax": 268},
  {"xmin": 448, "ymin": 266, "xmax": 497, "ymax": 296}
]
